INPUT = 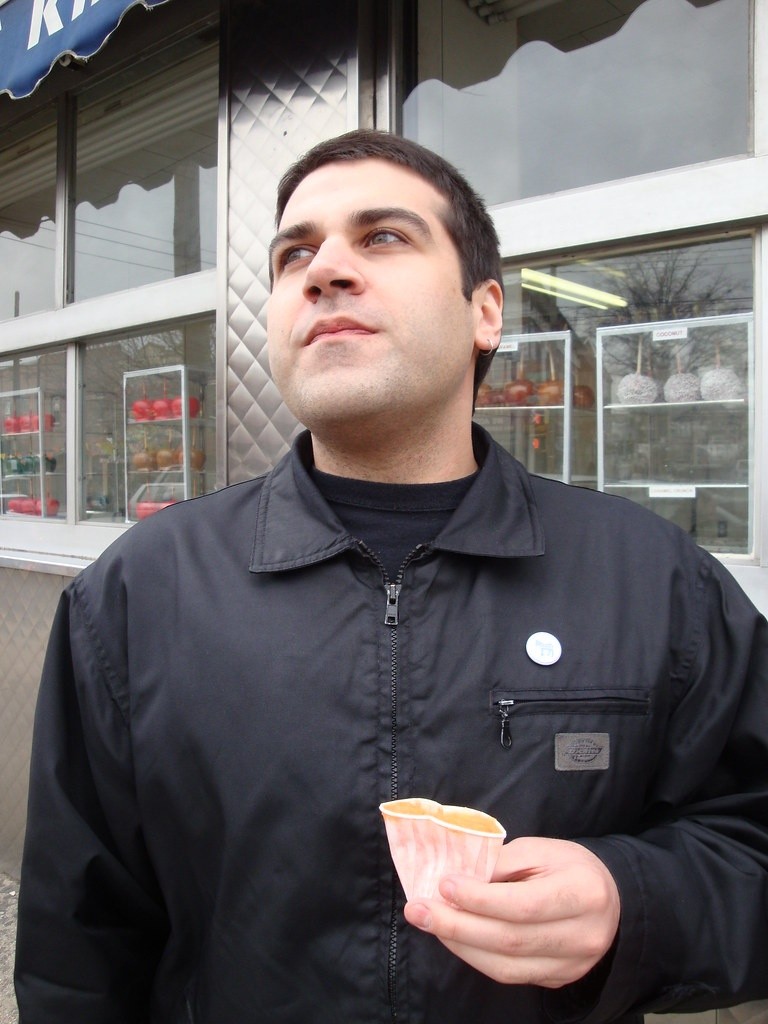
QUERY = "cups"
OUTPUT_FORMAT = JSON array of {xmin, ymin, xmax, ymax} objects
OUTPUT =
[{"xmin": 379, "ymin": 795, "xmax": 507, "ymax": 910}]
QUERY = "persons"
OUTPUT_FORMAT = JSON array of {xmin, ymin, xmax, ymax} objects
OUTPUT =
[{"xmin": 13, "ymin": 129, "xmax": 767, "ymax": 1024}]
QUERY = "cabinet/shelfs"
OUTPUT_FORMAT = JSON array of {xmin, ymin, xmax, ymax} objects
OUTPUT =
[
  {"xmin": 475, "ymin": 330, "xmax": 628, "ymax": 484},
  {"xmin": 123, "ymin": 364, "xmax": 216, "ymax": 526},
  {"xmin": 0, "ymin": 387, "xmax": 65, "ymax": 520},
  {"xmin": 593, "ymin": 312, "xmax": 757, "ymax": 565}
]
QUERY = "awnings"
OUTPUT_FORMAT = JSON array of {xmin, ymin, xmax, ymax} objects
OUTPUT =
[{"xmin": 0, "ymin": 0, "xmax": 173, "ymax": 101}]
